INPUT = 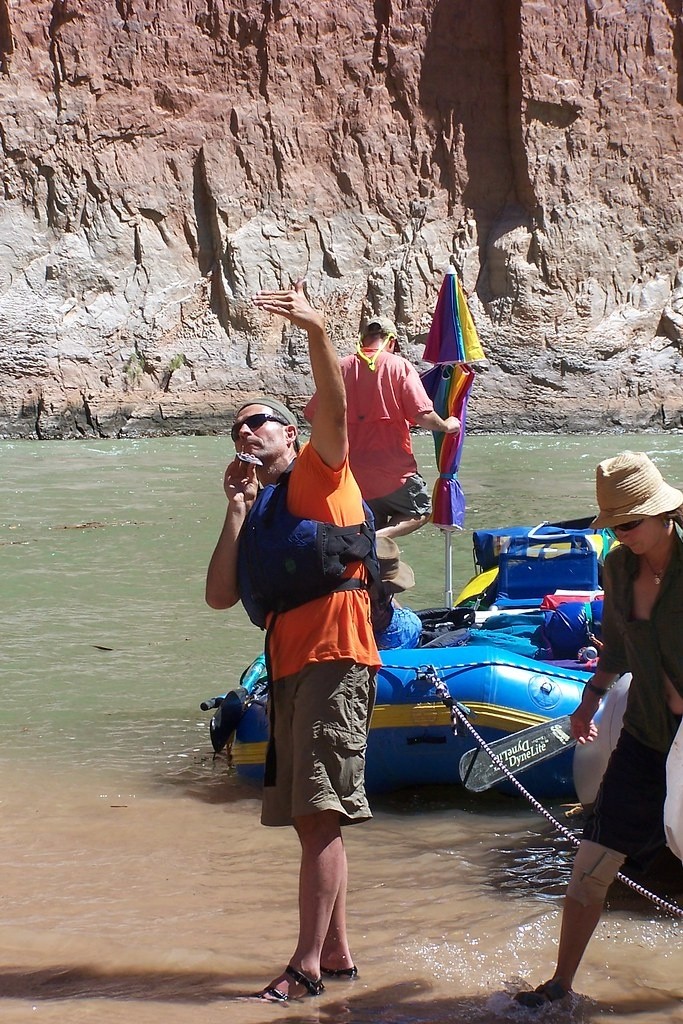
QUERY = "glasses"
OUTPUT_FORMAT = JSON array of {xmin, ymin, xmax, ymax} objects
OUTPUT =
[
  {"xmin": 610, "ymin": 519, "xmax": 644, "ymax": 533},
  {"xmin": 231, "ymin": 414, "xmax": 288, "ymax": 442}
]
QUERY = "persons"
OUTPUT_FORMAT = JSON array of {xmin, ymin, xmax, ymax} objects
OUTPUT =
[
  {"xmin": 303, "ymin": 314, "xmax": 461, "ymax": 649},
  {"xmin": 205, "ymin": 279, "xmax": 384, "ymax": 1008},
  {"xmin": 512, "ymin": 450, "xmax": 682, "ymax": 1011}
]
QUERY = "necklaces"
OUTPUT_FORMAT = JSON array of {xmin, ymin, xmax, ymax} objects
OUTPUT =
[{"xmin": 643, "ymin": 543, "xmax": 673, "ymax": 585}]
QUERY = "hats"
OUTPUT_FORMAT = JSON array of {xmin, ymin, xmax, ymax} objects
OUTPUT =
[
  {"xmin": 589, "ymin": 452, "xmax": 683, "ymax": 529},
  {"xmin": 376, "ymin": 536, "xmax": 416, "ymax": 593},
  {"xmin": 362, "ymin": 317, "xmax": 401, "ymax": 352},
  {"xmin": 235, "ymin": 398, "xmax": 299, "ymax": 453}
]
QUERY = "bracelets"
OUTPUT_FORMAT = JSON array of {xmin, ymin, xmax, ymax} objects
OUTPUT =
[{"xmin": 587, "ymin": 676, "xmax": 609, "ymax": 697}]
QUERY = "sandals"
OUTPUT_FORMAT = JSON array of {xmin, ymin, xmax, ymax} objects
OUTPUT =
[
  {"xmin": 514, "ymin": 980, "xmax": 572, "ymax": 1009},
  {"xmin": 255, "ymin": 965, "xmax": 326, "ymax": 1003},
  {"xmin": 320, "ymin": 965, "xmax": 357, "ymax": 978}
]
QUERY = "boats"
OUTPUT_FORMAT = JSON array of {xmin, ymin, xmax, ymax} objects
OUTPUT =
[
  {"xmin": 570, "ymin": 672, "xmax": 683, "ymax": 871},
  {"xmin": 195, "ymin": 264, "xmax": 618, "ymax": 800}
]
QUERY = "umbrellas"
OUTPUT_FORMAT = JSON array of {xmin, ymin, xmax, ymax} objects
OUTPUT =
[{"xmin": 417, "ymin": 266, "xmax": 490, "ymax": 613}]
